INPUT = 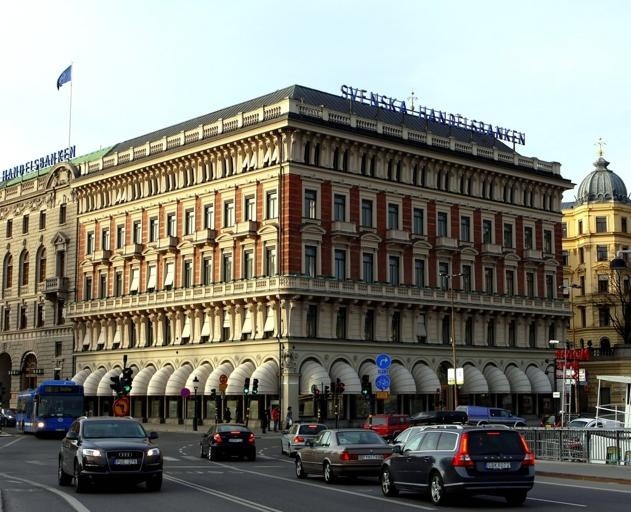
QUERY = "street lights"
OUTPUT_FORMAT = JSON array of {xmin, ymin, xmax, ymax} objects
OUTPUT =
[
  {"xmin": 438, "ymin": 271, "xmax": 465, "ymax": 410},
  {"xmin": 557, "ymin": 282, "xmax": 583, "ymax": 415},
  {"xmin": 192, "ymin": 377, "xmax": 199, "ymax": 432},
  {"xmin": 547, "ymin": 340, "xmax": 569, "ymax": 431}
]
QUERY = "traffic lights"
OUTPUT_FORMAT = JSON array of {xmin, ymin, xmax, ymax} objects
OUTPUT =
[
  {"xmin": 119, "ymin": 368, "xmax": 133, "ymax": 393},
  {"xmin": 109, "ymin": 376, "xmax": 119, "ymax": 393},
  {"xmin": 252, "ymin": 379, "xmax": 259, "ymax": 394},
  {"xmin": 243, "ymin": 377, "xmax": 250, "ymax": 394},
  {"xmin": 361, "ymin": 375, "xmax": 372, "ymax": 400}
]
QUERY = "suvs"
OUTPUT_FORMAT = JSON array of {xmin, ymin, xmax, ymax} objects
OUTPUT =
[
  {"xmin": 57, "ymin": 415, "xmax": 164, "ymax": 491},
  {"xmin": 362, "ymin": 414, "xmax": 411, "ymax": 439}
]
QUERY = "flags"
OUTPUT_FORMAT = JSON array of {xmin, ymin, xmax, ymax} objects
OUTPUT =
[{"xmin": 57, "ymin": 64, "xmax": 72, "ymax": 90}]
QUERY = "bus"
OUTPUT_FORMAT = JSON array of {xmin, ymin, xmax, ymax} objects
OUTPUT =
[{"xmin": 14, "ymin": 379, "xmax": 84, "ymax": 436}]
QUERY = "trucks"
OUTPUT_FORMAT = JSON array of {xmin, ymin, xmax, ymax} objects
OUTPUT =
[{"xmin": 455, "ymin": 405, "xmax": 527, "ymax": 427}]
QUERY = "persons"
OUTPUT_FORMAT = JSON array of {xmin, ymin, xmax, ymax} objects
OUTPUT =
[
  {"xmin": 223, "ymin": 407, "xmax": 231, "ymax": 423},
  {"xmin": 260, "ymin": 406, "xmax": 280, "ymax": 433},
  {"xmin": 0, "ymin": 383, "xmax": 11, "ymax": 409},
  {"xmin": 56, "ymin": 399, "xmax": 65, "ymax": 411},
  {"xmin": 540, "ymin": 414, "xmax": 550, "ymax": 427},
  {"xmin": 235, "ymin": 407, "xmax": 243, "ymax": 423},
  {"xmin": 553, "ymin": 416, "xmax": 561, "ymax": 427},
  {"xmin": 286, "ymin": 406, "xmax": 293, "ymax": 430},
  {"xmin": 214, "ymin": 408, "xmax": 219, "ymax": 423}
]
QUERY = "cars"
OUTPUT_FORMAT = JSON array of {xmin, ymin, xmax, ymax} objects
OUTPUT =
[
  {"xmin": 281, "ymin": 422, "xmax": 329, "ymax": 458},
  {"xmin": 541, "ymin": 414, "xmax": 603, "ymax": 428},
  {"xmin": 0, "ymin": 408, "xmax": 16, "ymax": 428},
  {"xmin": 379, "ymin": 425, "xmax": 536, "ymax": 506},
  {"xmin": 409, "ymin": 410, "xmax": 468, "ymax": 427},
  {"xmin": 199, "ymin": 423, "xmax": 256, "ymax": 460},
  {"xmin": 294, "ymin": 428, "xmax": 393, "ymax": 485}
]
